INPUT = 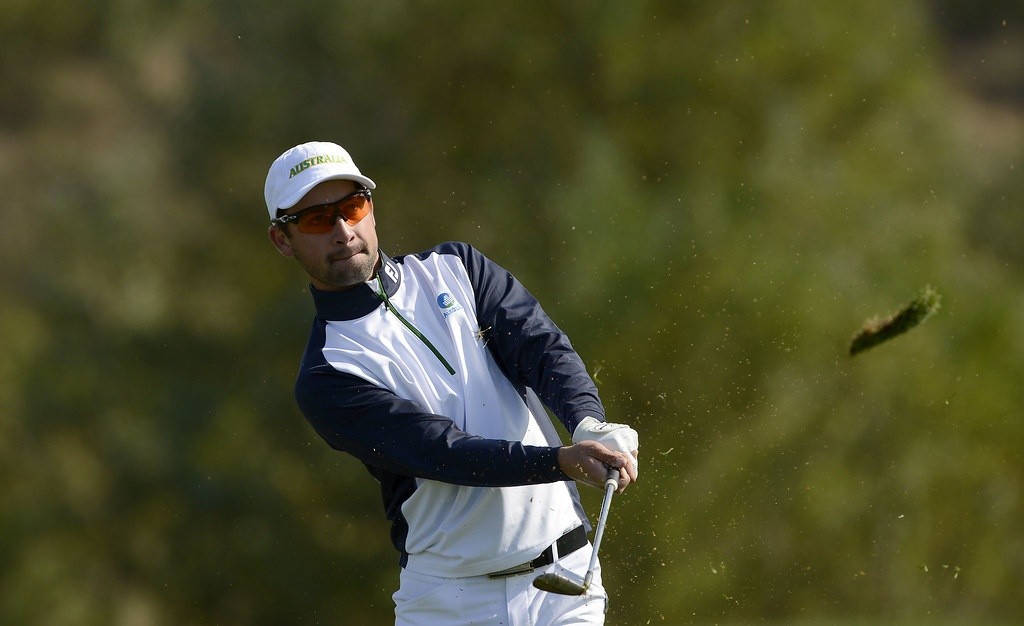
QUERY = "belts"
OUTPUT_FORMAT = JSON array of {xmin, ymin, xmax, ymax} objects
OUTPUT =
[{"xmin": 489, "ymin": 524, "xmax": 589, "ymax": 579}]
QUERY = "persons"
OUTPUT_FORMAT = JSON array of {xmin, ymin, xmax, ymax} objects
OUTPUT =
[{"xmin": 264, "ymin": 141, "xmax": 639, "ymax": 626}]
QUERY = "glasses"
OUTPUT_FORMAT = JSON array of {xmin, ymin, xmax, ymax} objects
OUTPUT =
[{"xmin": 269, "ymin": 188, "xmax": 374, "ymax": 238}]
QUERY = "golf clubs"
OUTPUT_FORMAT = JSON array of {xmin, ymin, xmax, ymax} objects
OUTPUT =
[{"xmin": 530, "ymin": 463, "xmax": 620, "ymax": 601}]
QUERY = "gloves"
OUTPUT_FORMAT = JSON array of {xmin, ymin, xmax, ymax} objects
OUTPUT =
[{"xmin": 572, "ymin": 417, "xmax": 637, "ymax": 481}]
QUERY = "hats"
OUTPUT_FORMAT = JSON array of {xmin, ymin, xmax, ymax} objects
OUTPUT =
[{"xmin": 263, "ymin": 141, "xmax": 377, "ymax": 222}]
